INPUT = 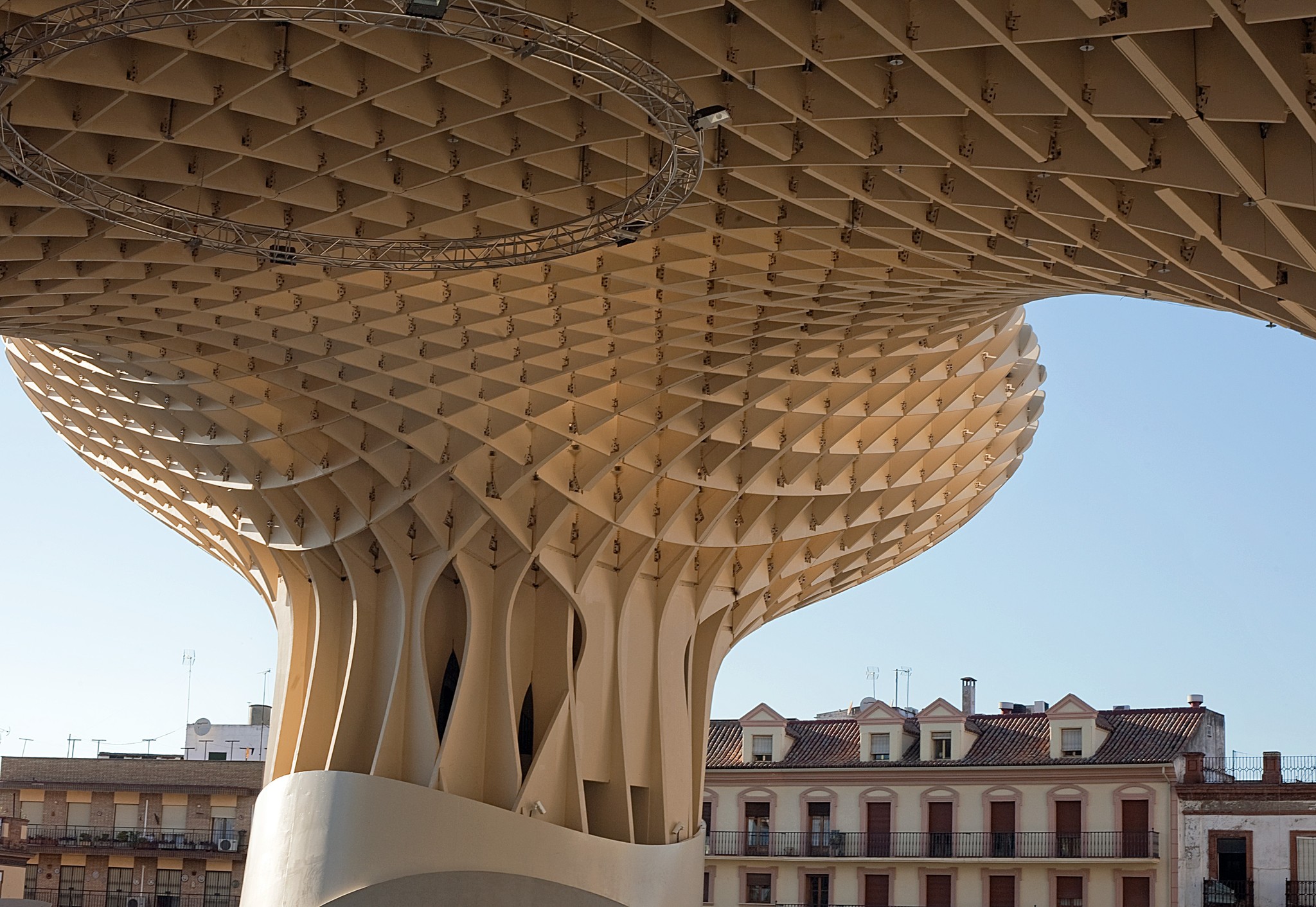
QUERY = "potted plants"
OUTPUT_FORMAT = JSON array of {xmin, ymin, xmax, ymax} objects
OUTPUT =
[{"xmin": 29, "ymin": 830, "xmax": 215, "ymax": 850}]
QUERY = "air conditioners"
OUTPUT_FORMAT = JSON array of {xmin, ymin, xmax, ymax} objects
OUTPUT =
[{"xmin": 218, "ymin": 839, "xmax": 238, "ymax": 851}]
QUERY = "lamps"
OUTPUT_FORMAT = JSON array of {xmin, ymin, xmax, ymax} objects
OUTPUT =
[
  {"xmin": 688, "ymin": 105, "xmax": 731, "ymax": 133},
  {"xmin": 612, "ymin": 220, "xmax": 647, "ymax": 247},
  {"xmin": 405, "ymin": 0, "xmax": 449, "ymax": 19},
  {"xmin": 0, "ymin": 168, "xmax": 27, "ymax": 187},
  {"xmin": 270, "ymin": 244, "xmax": 296, "ymax": 266}
]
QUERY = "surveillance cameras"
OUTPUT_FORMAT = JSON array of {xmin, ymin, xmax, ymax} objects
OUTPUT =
[
  {"xmin": 672, "ymin": 821, "xmax": 684, "ymax": 834},
  {"xmin": 534, "ymin": 800, "xmax": 546, "ymax": 814}
]
